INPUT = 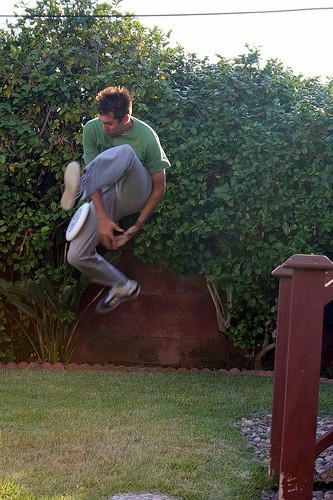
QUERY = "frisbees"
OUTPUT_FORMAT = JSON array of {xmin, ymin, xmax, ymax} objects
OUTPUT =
[{"xmin": 65, "ymin": 203, "xmax": 91, "ymax": 241}]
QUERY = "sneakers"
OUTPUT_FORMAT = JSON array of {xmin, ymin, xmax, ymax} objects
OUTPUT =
[
  {"xmin": 95, "ymin": 279, "xmax": 141, "ymax": 314},
  {"xmin": 60, "ymin": 161, "xmax": 84, "ymax": 211}
]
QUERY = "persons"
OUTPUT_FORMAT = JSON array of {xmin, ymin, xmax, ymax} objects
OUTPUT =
[{"xmin": 60, "ymin": 86, "xmax": 171, "ymax": 313}]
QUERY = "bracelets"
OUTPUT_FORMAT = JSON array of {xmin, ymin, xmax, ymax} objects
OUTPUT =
[{"xmin": 134, "ymin": 220, "xmax": 144, "ymax": 229}]
